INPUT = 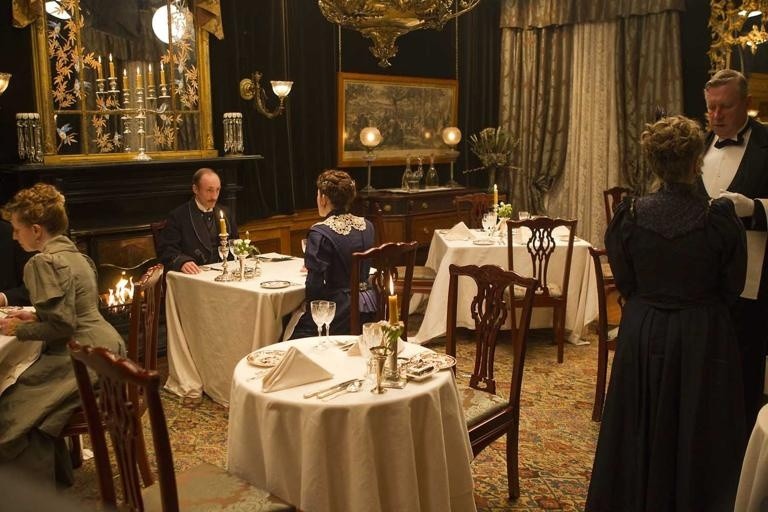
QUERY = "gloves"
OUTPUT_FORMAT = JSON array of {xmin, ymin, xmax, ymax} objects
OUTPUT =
[{"xmin": 716, "ymin": 187, "xmax": 755, "ymax": 220}]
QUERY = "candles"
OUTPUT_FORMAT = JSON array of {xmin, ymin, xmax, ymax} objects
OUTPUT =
[
  {"xmin": 123, "ymin": 68, "xmax": 129, "ymax": 90},
  {"xmin": 159, "ymin": 60, "xmax": 166, "ymax": 84},
  {"xmin": 108, "ymin": 53, "xmax": 115, "ymax": 77},
  {"xmin": 147, "ymin": 63, "xmax": 154, "ymax": 85},
  {"xmin": 136, "ymin": 66, "xmax": 143, "ymax": 88},
  {"xmin": 96, "ymin": 55, "xmax": 103, "ymax": 79}
]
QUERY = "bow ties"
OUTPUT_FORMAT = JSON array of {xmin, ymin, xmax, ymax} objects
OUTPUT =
[
  {"xmin": 713, "ymin": 133, "xmax": 746, "ymax": 150},
  {"xmin": 200, "ymin": 212, "xmax": 216, "ymax": 220}
]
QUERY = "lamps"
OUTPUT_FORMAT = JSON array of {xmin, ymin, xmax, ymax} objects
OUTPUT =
[
  {"xmin": 359, "ymin": 126, "xmax": 382, "ymax": 192},
  {"xmin": 442, "ymin": 126, "xmax": 462, "ymax": 188},
  {"xmin": 151, "ymin": 3, "xmax": 187, "ymax": 43},
  {"xmin": 240, "ymin": 70, "xmax": 293, "ymax": 120},
  {"xmin": 0, "ymin": 71, "xmax": 13, "ymax": 96}
]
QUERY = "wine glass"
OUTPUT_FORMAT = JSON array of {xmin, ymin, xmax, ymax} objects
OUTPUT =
[
  {"xmin": 217, "ymin": 240, "xmax": 242, "ymax": 280},
  {"xmin": 482, "ymin": 211, "xmax": 542, "ymax": 244},
  {"xmin": 402, "ymin": 153, "xmax": 439, "ymax": 191},
  {"xmin": 309, "ymin": 299, "xmax": 336, "ymax": 350},
  {"xmin": 360, "ymin": 323, "xmax": 382, "ymax": 386}
]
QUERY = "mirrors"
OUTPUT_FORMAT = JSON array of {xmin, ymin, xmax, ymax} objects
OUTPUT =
[{"xmin": 30, "ymin": 0, "xmax": 219, "ymax": 167}]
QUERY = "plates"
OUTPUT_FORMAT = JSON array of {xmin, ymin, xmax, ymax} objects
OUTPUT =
[
  {"xmin": 246, "ymin": 349, "xmax": 289, "ymax": 367},
  {"xmin": 472, "ymin": 239, "xmax": 494, "ymax": 245},
  {"xmin": 259, "ymin": 280, "xmax": 291, "ymax": 289},
  {"xmin": 559, "ymin": 238, "xmax": 582, "ymax": 242},
  {"xmin": 394, "ymin": 353, "xmax": 456, "ymax": 382}
]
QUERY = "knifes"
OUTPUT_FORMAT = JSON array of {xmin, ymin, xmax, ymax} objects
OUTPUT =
[{"xmin": 302, "ymin": 379, "xmax": 364, "ymax": 400}]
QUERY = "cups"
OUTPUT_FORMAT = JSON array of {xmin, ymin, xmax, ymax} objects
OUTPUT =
[
  {"xmin": 243, "ymin": 257, "xmax": 258, "ymax": 279},
  {"xmin": 221, "ymin": 111, "xmax": 245, "ymax": 154},
  {"xmin": 16, "ymin": 113, "xmax": 43, "ymax": 163}
]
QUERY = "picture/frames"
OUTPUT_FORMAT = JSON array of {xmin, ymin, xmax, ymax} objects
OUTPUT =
[{"xmin": 337, "ymin": 72, "xmax": 459, "ymax": 167}]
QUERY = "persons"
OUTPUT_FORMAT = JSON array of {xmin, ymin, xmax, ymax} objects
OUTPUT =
[
  {"xmin": 693, "ymin": 68, "xmax": 767, "ymax": 454},
  {"xmin": 0, "ymin": 180, "xmax": 129, "ymax": 487},
  {"xmin": 0, "ymin": 220, "xmax": 36, "ymax": 307},
  {"xmin": 279, "ymin": 168, "xmax": 378, "ymax": 339},
  {"xmin": 583, "ymin": 113, "xmax": 745, "ymax": 512},
  {"xmin": 156, "ymin": 166, "xmax": 238, "ymax": 277}
]
